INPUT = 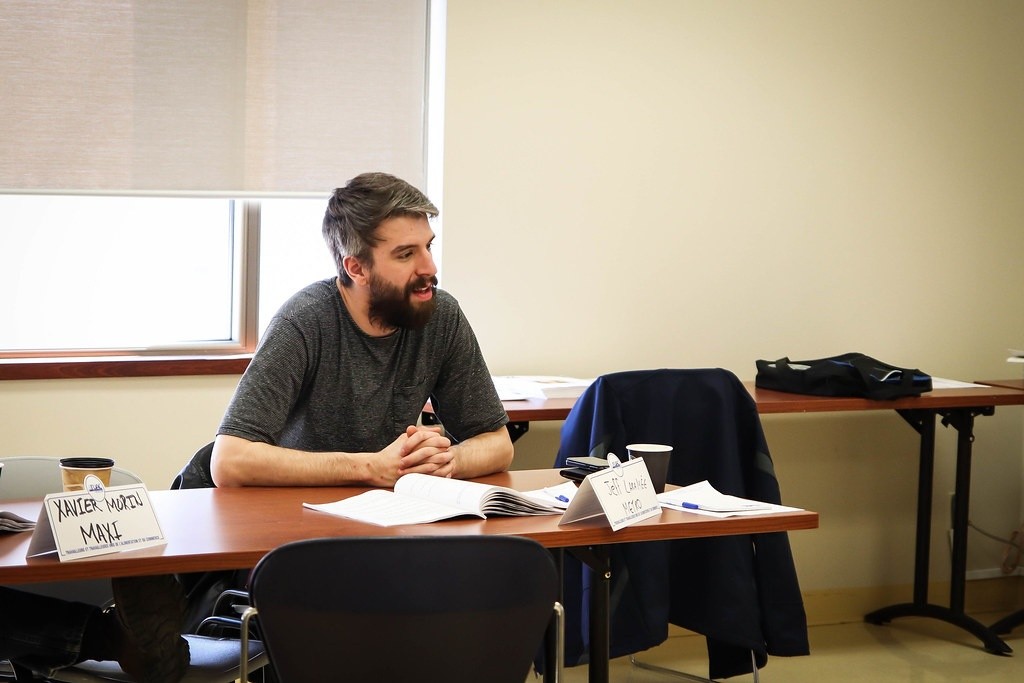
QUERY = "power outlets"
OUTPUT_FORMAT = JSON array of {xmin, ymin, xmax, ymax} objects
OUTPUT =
[{"xmin": 947, "ymin": 491, "xmax": 975, "ymax": 529}]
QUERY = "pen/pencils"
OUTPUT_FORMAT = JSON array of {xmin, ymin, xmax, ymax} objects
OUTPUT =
[
  {"xmin": 660, "ymin": 499, "xmax": 699, "ymax": 510},
  {"xmin": 543, "ymin": 487, "xmax": 569, "ymax": 503}
]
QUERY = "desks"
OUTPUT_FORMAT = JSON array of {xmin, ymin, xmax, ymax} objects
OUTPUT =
[
  {"xmin": 0, "ymin": 468, "xmax": 822, "ymax": 683},
  {"xmin": 421, "ymin": 374, "xmax": 1024, "ymax": 658}
]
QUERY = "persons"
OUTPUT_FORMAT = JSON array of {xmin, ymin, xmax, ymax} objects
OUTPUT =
[{"xmin": 208, "ymin": 172, "xmax": 515, "ymax": 489}]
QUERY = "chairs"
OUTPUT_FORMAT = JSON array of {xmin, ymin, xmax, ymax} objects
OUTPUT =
[
  {"xmin": 526, "ymin": 367, "xmax": 810, "ymax": 683},
  {"xmin": 241, "ymin": 534, "xmax": 564, "ymax": 683},
  {"xmin": 50, "ymin": 586, "xmax": 268, "ymax": 683}
]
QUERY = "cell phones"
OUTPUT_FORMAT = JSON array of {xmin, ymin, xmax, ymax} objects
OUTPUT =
[{"xmin": 565, "ymin": 456, "xmax": 610, "ymax": 472}]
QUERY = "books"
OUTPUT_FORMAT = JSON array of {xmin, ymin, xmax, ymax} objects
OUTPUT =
[
  {"xmin": 0, "ymin": 510, "xmax": 37, "ymax": 533},
  {"xmin": 301, "ymin": 471, "xmax": 566, "ymax": 527}
]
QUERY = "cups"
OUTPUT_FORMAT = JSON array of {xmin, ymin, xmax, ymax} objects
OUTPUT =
[
  {"xmin": 627, "ymin": 444, "xmax": 673, "ymax": 494},
  {"xmin": 59, "ymin": 458, "xmax": 114, "ymax": 492}
]
